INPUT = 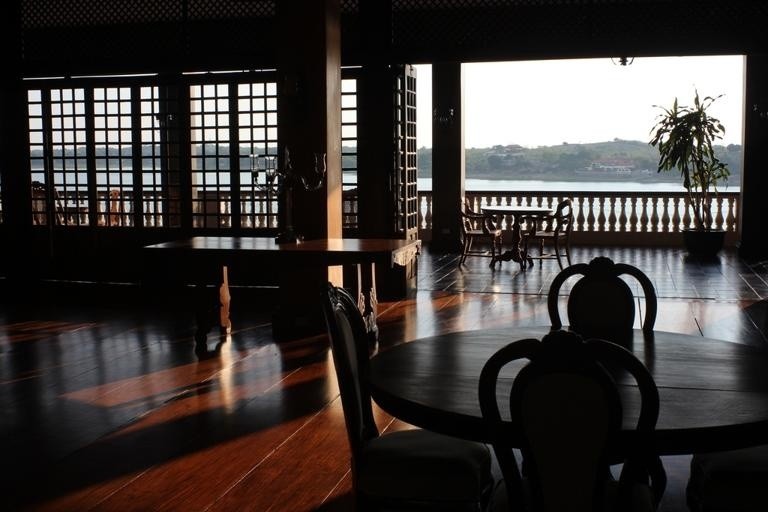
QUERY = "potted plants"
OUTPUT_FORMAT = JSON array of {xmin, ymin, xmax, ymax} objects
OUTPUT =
[{"xmin": 645, "ymin": 89, "xmax": 734, "ymax": 266}]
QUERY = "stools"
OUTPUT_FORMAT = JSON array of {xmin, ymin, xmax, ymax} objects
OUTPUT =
[{"xmin": 681, "ymin": 443, "xmax": 768, "ymax": 511}]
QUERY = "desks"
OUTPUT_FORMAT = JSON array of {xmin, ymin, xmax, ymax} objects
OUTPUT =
[
  {"xmin": 142, "ymin": 233, "xmax": 423, "ymax": 354},
  {"xmin": 367, "ymin": 327, "xmax": 768, "ymax": 511}
]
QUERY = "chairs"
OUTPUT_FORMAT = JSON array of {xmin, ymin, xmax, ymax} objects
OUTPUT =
[
  {"xmin": 548, "ymin": 257, "xmax": 658, "ymax": 333},
  {"xmin": 30, "ymin": 181, "xmax": 86, "ymax": 226},
  {"xmin": 456, "ymin": 195, "xmax": 578, "ymax": 273},
  {"xmin": 477, "ymin": 326, "xmax": 668, "ymax": 511},
  {"xmin": 319, "ymin": 284, "xmax": 495, "ymax": 511}
]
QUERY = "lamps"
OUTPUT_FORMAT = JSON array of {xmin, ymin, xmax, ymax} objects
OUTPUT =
[
  {"xmin": 249, "ymin": 146, "xmax": 327, "ymax": 245},
  {"xmin": 432, "ymin": 103, "xmax": 457, "ymax": 131}
]
